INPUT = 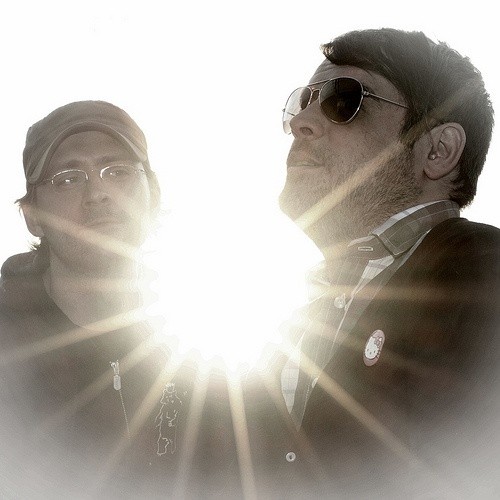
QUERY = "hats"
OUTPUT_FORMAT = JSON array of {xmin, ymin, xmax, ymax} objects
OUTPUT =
[{"xmin": 23, "ymin": 99, "xmax": 149, "ymax": 185}]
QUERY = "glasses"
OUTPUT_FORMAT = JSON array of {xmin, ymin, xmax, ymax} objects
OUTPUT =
[
  {"xmin": 31, "ymin": 164, "xmax": 150, "ymax": 192},
  {"xmin": 282, "ymin": 77, "xmax": 439, "ymax": 134}
]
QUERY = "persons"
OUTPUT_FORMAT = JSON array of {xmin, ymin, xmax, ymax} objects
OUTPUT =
[
  {"xmin": 269, "ymin": 28, "xmax": 498, "ymax": 500},
  {"xmin": 0, "ymin": 101, "xmax": 239, "ymax": 498}
]
